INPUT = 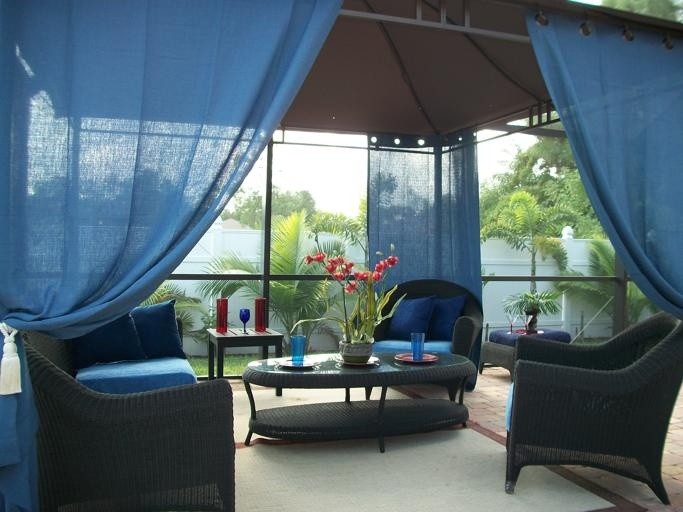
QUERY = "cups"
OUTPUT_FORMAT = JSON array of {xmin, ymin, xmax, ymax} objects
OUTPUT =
[
  {"xmin": 409, "ymin": 332, "xmax": 424, "ymax": 360},
  {"xmin": 215, "ymin": 299, "xmax": 228, "ymax": 334},
  {"xmin": 290, "ymin": 334, "xmax": 306, "ymax": 365},
  {"xmin": 253, "ymin": 297, "xmax": 266, "ymax": 333}
]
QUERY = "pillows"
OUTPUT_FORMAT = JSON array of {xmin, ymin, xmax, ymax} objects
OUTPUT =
[
  {"xmin": 380, "ymin": 294, "xmax": 436, "ymax": 341},
  {"xmin": 70, "ymin": 310, "xmax": 148, "ymax": 377},
  {"xmin": 130, "ymin": 297, "xmax": 188, "ymax": 359},
  {"xmin": 425, "ymin": 293, "xmax": 469, "ymax": 342}
]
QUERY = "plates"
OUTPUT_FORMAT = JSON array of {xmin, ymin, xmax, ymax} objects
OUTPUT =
[
  {"xmin": 336, "ymin": 356, "xmax": 379, "ymax": 366},
  {"xmin": 393, "ymin": 351, "xmax": 439, "ymax": 364},
  {"xmin": 276, "ymin": 358, "xmax": 315, "ymax": 369}
]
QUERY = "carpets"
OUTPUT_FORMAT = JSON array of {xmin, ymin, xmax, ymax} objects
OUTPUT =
[{"xmin": 196, "ymin": 386, "xmax": 652, "ymax": 512}]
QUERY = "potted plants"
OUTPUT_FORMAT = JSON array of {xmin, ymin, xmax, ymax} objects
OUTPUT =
[{"xmin": 501, "ymin": 286, "xmax": 570, "ymax": 334}]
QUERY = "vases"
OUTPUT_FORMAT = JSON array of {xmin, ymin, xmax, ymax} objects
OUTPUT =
[{"xmin": 339, "ymin": 340, "xmax": 374, "ymax": 363}]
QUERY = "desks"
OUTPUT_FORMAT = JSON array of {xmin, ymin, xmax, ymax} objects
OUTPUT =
[
  {"xmin": 478, "ymin": 328, "xmax": 571, "ymax": 383},
  {"xmin": 207, "ymin": 326, "xmax": 285, "ymax": 397}
]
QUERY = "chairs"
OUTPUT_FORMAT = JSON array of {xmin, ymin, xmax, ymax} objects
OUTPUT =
[
  {"xmin": 504, "ymin": 309, "xmax": 683, "ymax": 506},
  {"xmin": 20, "ymin": 316, "xmax": 236, "ymax": 512},
  {"xmin": 348, "ymin": 279, "xmax": 484, "ymax": 393}
]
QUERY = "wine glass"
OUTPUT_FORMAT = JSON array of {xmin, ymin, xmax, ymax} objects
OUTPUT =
[
  {"xmin": 506, "ymin": 314, "xmax": 518, "ymax": 334},
  {"xmin": 517, "ymin": 315, "xmax": 532, "ymax": 335},
  {"xmin": 239, "ymin": 309, "xmax": 250, "ymax": 334}
]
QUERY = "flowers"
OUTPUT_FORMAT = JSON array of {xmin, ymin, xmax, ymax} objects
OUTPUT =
[{"xmin": 289, "ymin": 243, "xmax": 408, "ymax": 344}]
